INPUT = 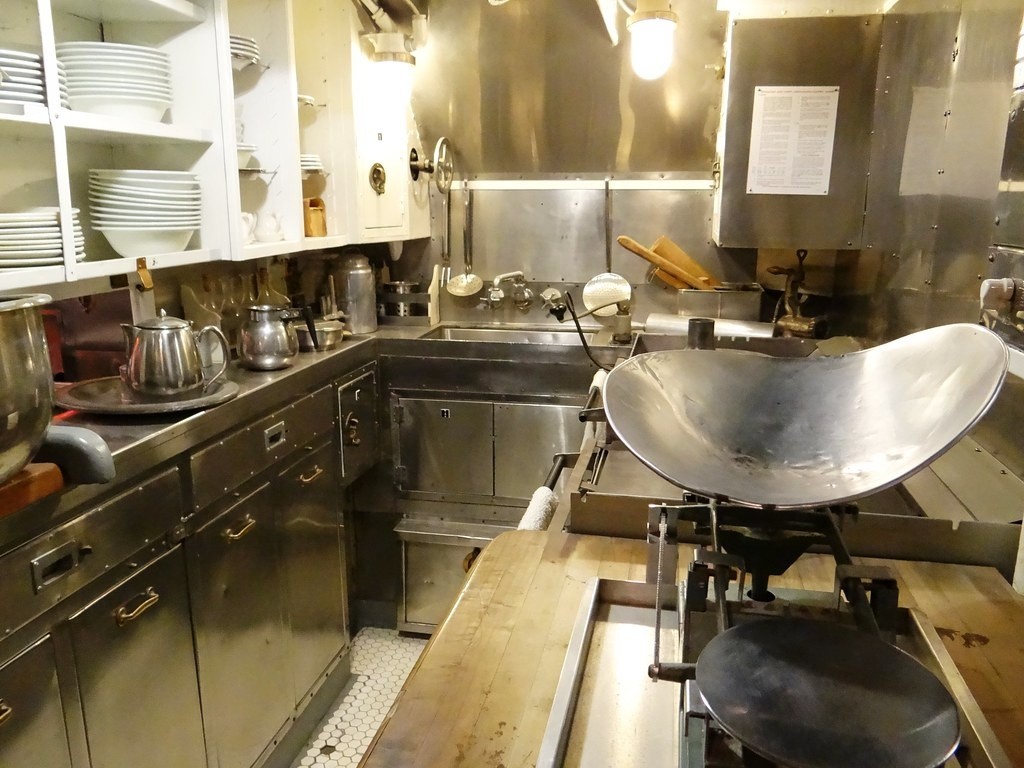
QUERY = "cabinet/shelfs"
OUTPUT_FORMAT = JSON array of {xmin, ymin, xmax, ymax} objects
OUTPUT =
[
  {"xmin": 279, "ymin": 440, "xmax": 345, "ymax": 708},
  {"xmin": 404, "ymin": 540, "xmax": 484, "ymax": 625},
  {"xmin": 0, "ymin": 328, "xmax": 382, "ymax": 767},
  {"xmin": 66, "ymin": 543, "xmax": 207, "ymax": 768},
  {"xmin": 397, "ymin": 397, "xmax": 585, "ymax": 500},
  {"xmin": 0, "ymin": 634, "xmax": 73, "ymax": 767},
  {"xmin": 711, "ymin": 13, "xmax": 884, "ymax": 250},
  {"xmin": 350, "ymin": 0, "xmax": 430, "ymax": 245},
  {"xmin": 216, "ymin": 0, "xmax": 362, "ymax": 262},
  {"xmin": 196, "ymin": 481, "xmax": 290, "ymax": 768},
  {"xmin": 0, "ymin": 0, "xmax": 229, "ymax": 291}
]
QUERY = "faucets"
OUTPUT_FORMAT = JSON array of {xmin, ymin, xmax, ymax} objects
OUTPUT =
[{"xmin": 490, "ymin": 271, "xmax": 525, "ymax": 300}]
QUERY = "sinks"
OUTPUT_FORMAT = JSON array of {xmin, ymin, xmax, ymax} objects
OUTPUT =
[{"xmin": 416, "ymin": 323, "xmax": 600, "ymax": 348}]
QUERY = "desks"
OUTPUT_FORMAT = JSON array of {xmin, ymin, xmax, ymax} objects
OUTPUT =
[{"xmin": 356, "ymin": 350, "xmax": 1023, "ymax": 768}]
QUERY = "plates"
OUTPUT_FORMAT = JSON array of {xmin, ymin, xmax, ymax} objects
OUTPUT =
[
  {"xmin": 298, "ymin": 94, "xmax": 315, "ymax": 102},
  {"xmin": 300, "ymin": 154, "xmax": 323, "ymax": 171},
  {"xmin": 0, "ymin": 49, "xmax": 72, "ymax": 115},
  {"xmin": 57, "ymin": 376, "xmax": 240, "ymax": 413},
  {"xmin": 0, "ymin": 205, "xmax": 86, "ymax": 267}
]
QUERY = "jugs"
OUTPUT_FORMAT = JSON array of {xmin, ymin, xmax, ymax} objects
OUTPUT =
[
  {"xmin": 237, "ymin": 304, "xmax": 299, "ymax": 370},
  {"xmin": 119, "ymin": 308, "xmax": 232, "ymax": 405}
]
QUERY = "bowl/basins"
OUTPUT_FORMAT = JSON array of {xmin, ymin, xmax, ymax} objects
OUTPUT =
[
  {"xmin": 294, "ymin": 321, "xmax": 343, "ymax": 352},
  {"xmin": 0, "ymin": 293, "xmax": 52, "ymax": 488},
  {"xmin": 86, "ymin": 168, "xmax": 202, "ymax": 257},
  {"xmin": 238, "ymin": 143, "xmax": 258, "ymax": 169},
  {"xmin": 229, "ymin": 34, "xmax": 258, "ymax": 71},
  {"xmin": 55, "ymin": 41, "xmax": 173, "ymax": 122}
]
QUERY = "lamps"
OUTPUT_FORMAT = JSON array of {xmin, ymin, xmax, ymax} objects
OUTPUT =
[
  {"xmin": 366, "ymin": 30, "xmax": 417, "ymax": 121},
  {"xmin": 625, "ymin": 2, "xmax": 680, "ymax": 84}
]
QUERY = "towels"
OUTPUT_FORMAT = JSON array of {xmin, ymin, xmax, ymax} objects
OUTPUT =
[{"xmin": 513, "ymin": 486, "xmax": 559, "ymax": 531}]
